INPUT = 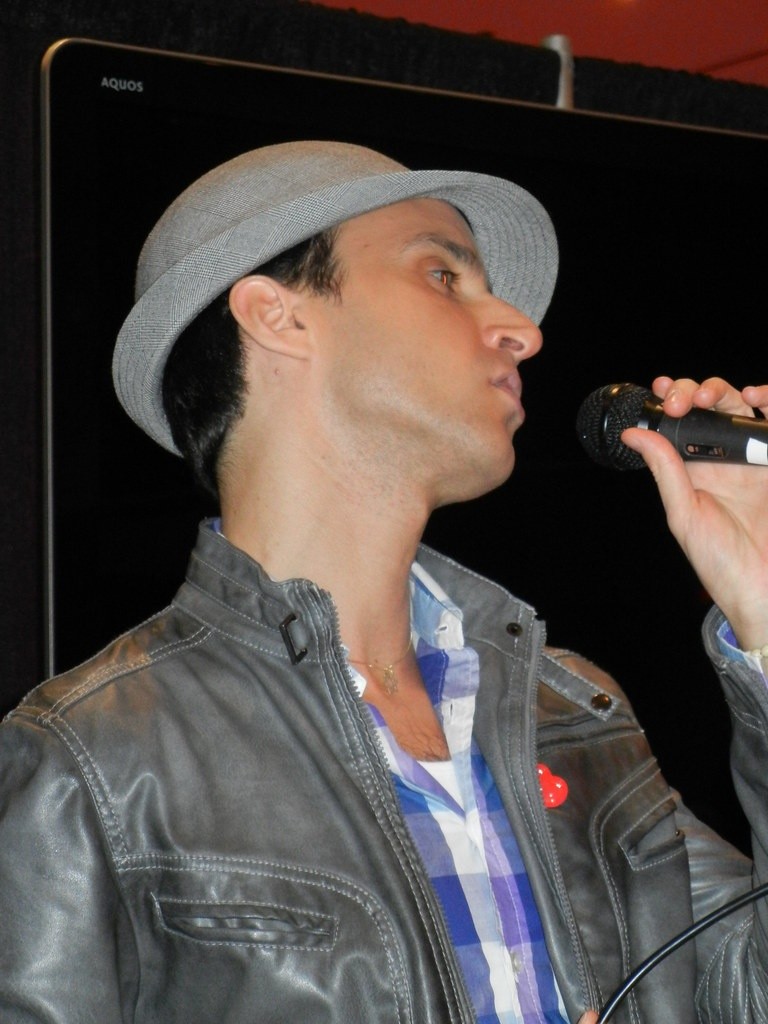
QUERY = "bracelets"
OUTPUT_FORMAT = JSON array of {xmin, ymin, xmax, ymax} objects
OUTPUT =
[{"xmin": 745, "ymin": 644, "xmax": 768, "ymax": 659}]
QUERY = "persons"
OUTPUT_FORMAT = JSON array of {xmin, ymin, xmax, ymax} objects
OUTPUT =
[{"xmin": 0, "ymin": 141, "xmax": 768, "ymax": 1024}]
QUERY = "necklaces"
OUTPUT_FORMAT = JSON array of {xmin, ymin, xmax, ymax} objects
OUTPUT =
[{"xmin": 349, "ymin": 635, "xmax": 412, "ymax": 696}]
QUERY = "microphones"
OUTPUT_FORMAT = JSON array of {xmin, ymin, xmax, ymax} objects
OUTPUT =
[{"xmin": 574, "ymin": 383, "xmax": 768, "ymax": 468}]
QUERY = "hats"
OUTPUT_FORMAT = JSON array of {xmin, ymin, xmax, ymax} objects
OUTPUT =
[{"xmin": 111, "ymin": 141, "xmax": 560, "ymax": 459}]
